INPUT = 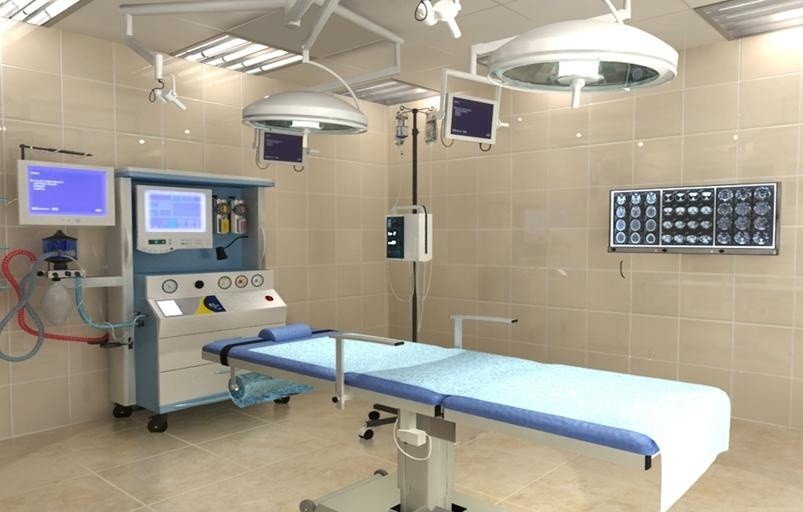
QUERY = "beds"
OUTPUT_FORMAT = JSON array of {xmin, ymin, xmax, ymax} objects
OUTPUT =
[{"xmin": 198, "ymin": 318, "xmax": 730, "ymax": 507}]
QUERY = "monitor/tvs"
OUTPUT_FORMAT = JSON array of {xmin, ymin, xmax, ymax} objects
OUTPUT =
[
  {"xmin": 17, "ymin": 160, "xmax": 115, "ymax": 226},
  {"xmin": 136, "ymin": 184, "xmax": 213, "ymax": 255},
  {"xmin": 258, "ymin": 130, "xmax": 307, "ymax": 167},
  {"xmin": 442, "ymin": 92, "xmax": 498, "ymax": 145}
]
